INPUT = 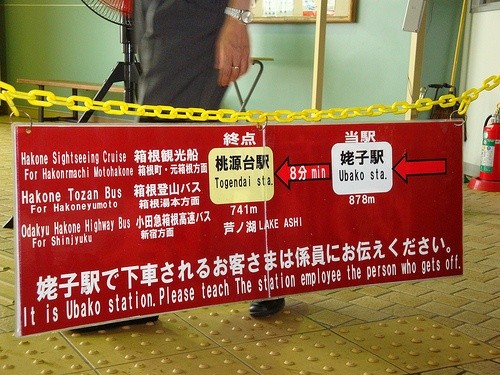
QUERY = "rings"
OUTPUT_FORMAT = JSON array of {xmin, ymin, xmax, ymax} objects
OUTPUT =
[{"xmin": 232, "ymin": 66, "xmax": 240, "ymax": 69}]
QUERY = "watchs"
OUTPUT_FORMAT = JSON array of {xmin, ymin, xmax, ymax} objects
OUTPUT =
[{"xmin": 223, "ymin": 6, "xmax": 255, "ymax": 24}]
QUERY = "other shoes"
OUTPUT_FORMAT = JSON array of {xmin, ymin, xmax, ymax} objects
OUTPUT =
[
  {"xmin": 73, "ymin": 316, "xmax": 158, "ymax": 332},
  {"xmin": 250, "ymin": 298, "xmax": 284, "ymax": 316}
]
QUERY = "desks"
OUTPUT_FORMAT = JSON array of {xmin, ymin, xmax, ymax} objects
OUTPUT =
[{"xmin": 232, "ymin": 56, "xmax": 276, "ymax": 123}]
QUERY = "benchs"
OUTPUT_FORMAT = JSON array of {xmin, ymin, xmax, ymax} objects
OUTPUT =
[{"xmin": 13, "ymin": 74, "xmax": 125, "ymax": 124}]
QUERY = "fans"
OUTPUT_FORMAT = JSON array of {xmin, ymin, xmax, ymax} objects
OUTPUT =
[{"xmin": 3, "ymin": 0, "xmax": 144, "ymax": 230}]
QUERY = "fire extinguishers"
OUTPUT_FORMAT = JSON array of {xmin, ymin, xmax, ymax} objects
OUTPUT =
[{"xmin": 479, "ymin": 113, "xmax": 500, "ymax": 182}]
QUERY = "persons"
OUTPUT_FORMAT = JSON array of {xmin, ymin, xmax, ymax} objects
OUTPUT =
[{"xmin": 70, "ymin": 0, "xmax": 286, "ymax": 334}]
